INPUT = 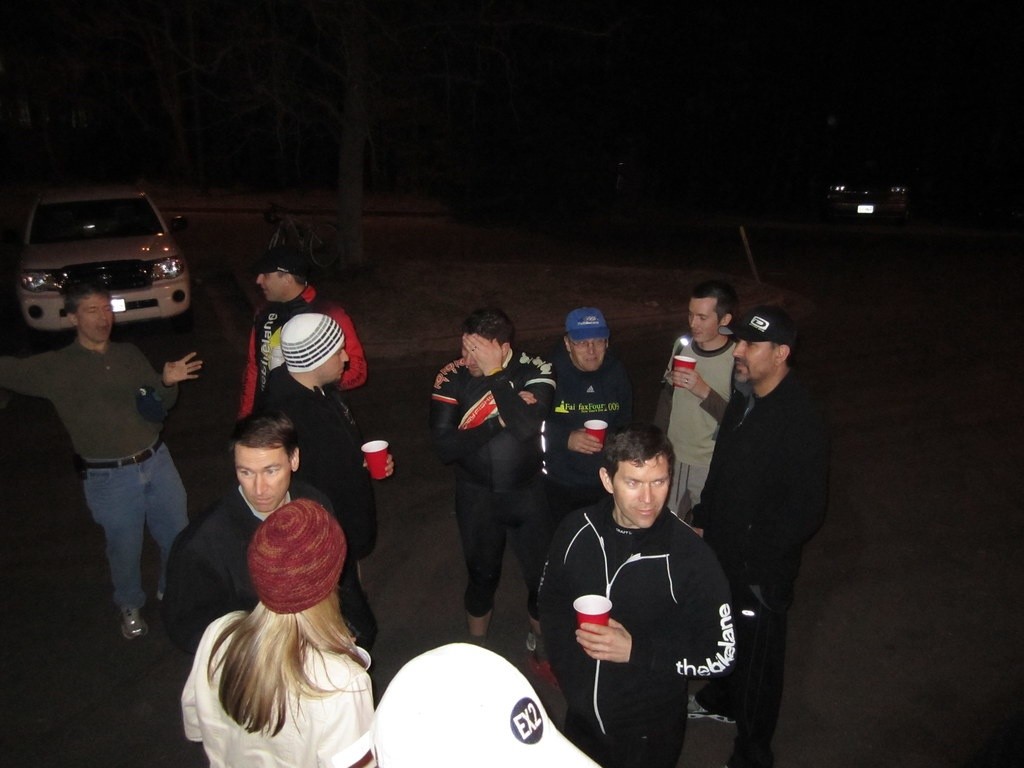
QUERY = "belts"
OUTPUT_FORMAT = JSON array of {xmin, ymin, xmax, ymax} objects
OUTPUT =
[{"xmin": 86, "ymin": 433, "xmax": 164, "ymax": 469}]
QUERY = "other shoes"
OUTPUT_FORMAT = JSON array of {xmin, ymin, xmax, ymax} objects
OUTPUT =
[
  {"xmin": 686, "ymin": 694, "xmax": 737, "ymax": 724},
  {"xmin": 529, "ymin": 654, "xmax": 563, "ymax": 692}
]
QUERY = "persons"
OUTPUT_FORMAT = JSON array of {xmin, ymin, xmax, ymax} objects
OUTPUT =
[
  {"xmin": 264, "ymin": 310, "xmax": 393, "ymax": 654},
  {"xmin": 159, "ymin": 407, "xmax": 378, "ymax": 670},
  {"xmin": 235, "ymin": 244, "xmax": 366, "ymax": 421},
  {"xmin": 178, "ymin": 499, "xmax": 379, "ymax": 768},
  {"xmin": 0, "ymin": 281, "xmax": 205, "ymax": 640},
  {"xmin": 427, "ymin": 306, "xmax": 554, "ymax": 640},
  {"xmin": 678, "ymin": 299, "xmax": 837, "ymax": 768},
  {"xmin": 530, "ymin": 423, "xmax": 736, "ymax": 768},
  {"xmin": 369, "ymin": 640, "xmax": 603, "ymax": 768},
  {"xmin": 651, "ymin": 277, "xmax": 743, "ymax": 517},
  {"xmin": 541, "ymin": 304, "xmax": 640, "ymax": 518}
]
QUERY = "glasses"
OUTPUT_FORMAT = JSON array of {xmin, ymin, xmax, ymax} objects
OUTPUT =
[{"xmin": 571, "ymin": 340, "xmax": 605, "ymax": 348}]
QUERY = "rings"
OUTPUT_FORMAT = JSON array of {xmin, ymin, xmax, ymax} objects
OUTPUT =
[
  {"xmin": 685, "ymin": 378, "xmax": 688, "ymax": 384},
  {"xmin": 472, "ymin": 346, "xmax": 477, "ymax": 351}
]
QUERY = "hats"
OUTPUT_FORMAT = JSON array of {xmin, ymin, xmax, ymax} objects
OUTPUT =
[
  {"xmin": 733, "ymin": 306, "xmax": 798, "ymax": 346},
  {"xmin": 565, "ymin": 307, "xmax": 611, "ymax": 341},
  {"xmin": 248, "ymin": 498, "xmax": 346, "ymax": 614},
  {"xmin": 371, "ymin": 641, "xmax": 599, "ymax": 768},
  {"xmin": 280, "ymin": 314, "xmax": 345, "ymax": 374},
  {"xmin": 251, "ymin": 245, "xmax": 304, "ymax": 276}
]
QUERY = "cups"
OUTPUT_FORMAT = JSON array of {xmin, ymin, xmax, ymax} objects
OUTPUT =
[
  {"xmin": 361, "ymin": 440, "xmax": 389, "ymax": 479},
  {"xmin": 573, "ymin": 594, "xmax": 612, "ymax": 650},
  {"xmin": 583, "ymin": 419, "xmax": 608, "ymax": 449},
  {"xmin": 674, "ymin": 356, "xmax": 696, "ymax": 388}
]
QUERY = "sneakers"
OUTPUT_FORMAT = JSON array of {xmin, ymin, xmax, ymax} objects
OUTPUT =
[
  {"xmin": 120, "ymin": 607, "xmax": 148, "ymax": 640},
  {"xmin": 156, "ymin": 590, "xmax": 165, "ymax": 599}
]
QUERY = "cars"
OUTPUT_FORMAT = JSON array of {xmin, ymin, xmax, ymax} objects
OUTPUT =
[
  {"xmin": 834, "ymin": 163, "xmax": 916, "ymax": 225},
  {"xmin": 17, "ymin": 181, "xmax": 197, "ymax": 341}
]
